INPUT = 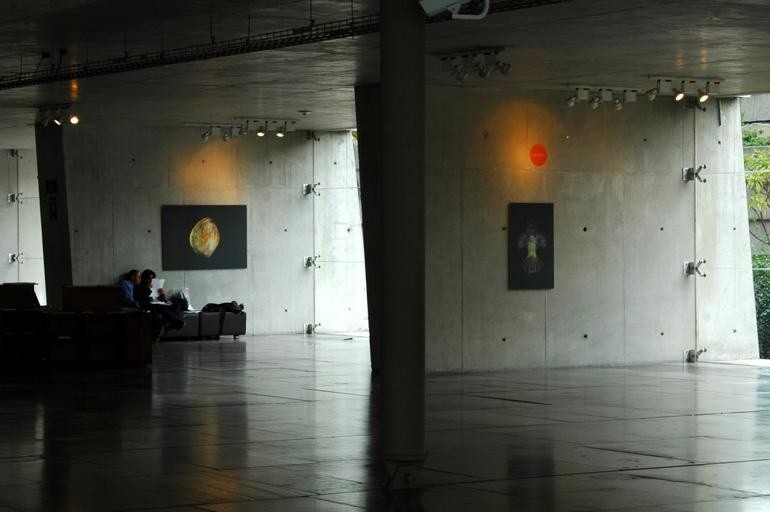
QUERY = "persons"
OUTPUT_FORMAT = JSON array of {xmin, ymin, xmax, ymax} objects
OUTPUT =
[
  {"xmin": 122, "ymin": 269, "xmax": 185, "ymax": 342},
  {"xmin": 518, "ymin": 221, "xmax": 546, "ymax": 274}
]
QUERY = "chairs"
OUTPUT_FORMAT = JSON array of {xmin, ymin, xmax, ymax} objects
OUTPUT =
[{"xmin": 1, "ymin": 281, "xmax": 245, "ymax": 369}]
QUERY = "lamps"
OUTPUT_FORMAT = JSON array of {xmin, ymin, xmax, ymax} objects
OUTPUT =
[
  {"xmin": 418, "ymin": 0, "xmax": 493, "ymax": 20},
  {"xmin": 563, "ymin": 74, "xmax": 723, "ymax": 111}
]
